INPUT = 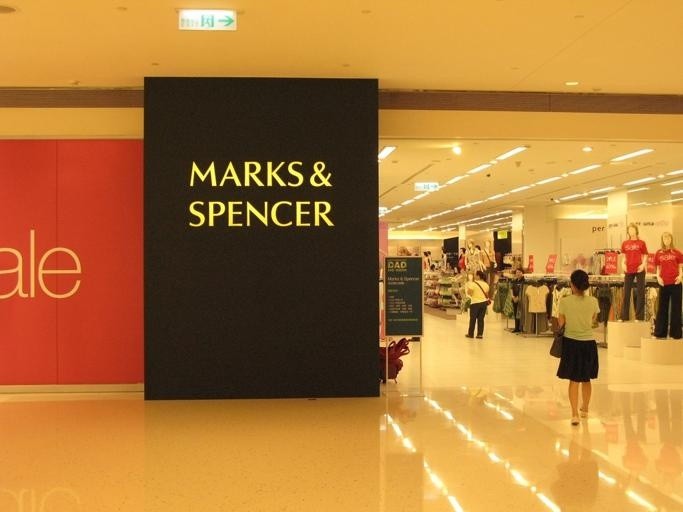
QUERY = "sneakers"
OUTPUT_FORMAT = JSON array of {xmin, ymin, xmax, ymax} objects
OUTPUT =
[
  {"xmin": 475, "ymin": 335, "xmax": 483, "ymax": 339},
  {"xmin": 571, "ymin": 416, "xmax": 580, "ymax": 426},
  {"xmin": 465, "ymin": 333, "xmax": 474, "ymax": 338},
  {"xmin": 579, "ymin": 407, "xmax": 588, "ymax": 417}
]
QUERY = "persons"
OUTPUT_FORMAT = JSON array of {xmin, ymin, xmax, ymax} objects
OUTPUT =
[
  {"xmin": 510, "ymin": 267, "xmax": 528, "ymax": 333},
  {"xmin": 652, "ymin": 232, "xmax": 683, "ymax": 340},
  {"xmin": 556, "ymin": 269, "xmax": 603, "ymax": 426},
  {"xmin": 464, "ymin": 270, "xmax": 490, "ymax": 339},
  {"xmin": 422, "ymin": 239, "xmax": 497, "ymax": 300},
  {"xmin": 615, "ymin": 223, "xmax": 649, "ymax": 324}
]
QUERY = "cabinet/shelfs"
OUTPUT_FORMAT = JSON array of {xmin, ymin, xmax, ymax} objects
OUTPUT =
[{"xmin": 422, "ymin": 271, "xmax": 443, "ymax": 309}]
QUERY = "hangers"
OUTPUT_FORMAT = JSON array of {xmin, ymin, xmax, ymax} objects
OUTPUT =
[{"xmin": 525, "ymin": 278, "xmax": 571, "ymax": 288}]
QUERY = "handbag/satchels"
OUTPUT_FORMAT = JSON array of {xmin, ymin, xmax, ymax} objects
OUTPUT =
[
  {"xmin": 489, "ymin": 261, "xmax": 494, "ymax": 268},
  {"xmin": 548, "ymin": 326, "xmax": 566, "ymax": 358},
  {"xmin": 486, "ymin": 297, "xmax": 491, "ymax": 305}
]
